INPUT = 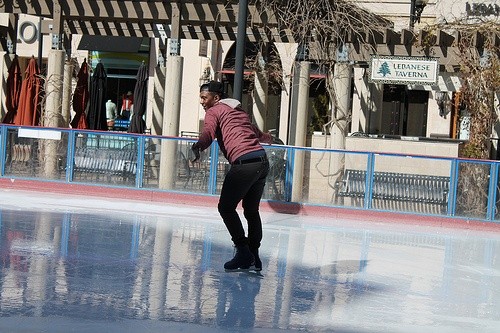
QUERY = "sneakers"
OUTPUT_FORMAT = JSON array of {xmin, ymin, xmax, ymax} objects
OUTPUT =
[
  {"xmin": 223, "ymin": 245, "xmax": 255, "ymax": 271},
  {"xmin": 245, "ymin": 244, "xmax": 264, "ymax": 279}
]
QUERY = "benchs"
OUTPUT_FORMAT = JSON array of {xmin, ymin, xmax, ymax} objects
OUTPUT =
[
  {"xmin": 338, "ymin": 168, "xmax": 452, "ymax": 215},
  {"xmin": 71, "ymin": 142, "xmax": 149, "ymax": 183}
]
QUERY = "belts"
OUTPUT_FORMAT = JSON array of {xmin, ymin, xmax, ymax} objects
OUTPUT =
[{"xmin": 234, "ymin": 157, "xmax": 268, "ymax": 165}]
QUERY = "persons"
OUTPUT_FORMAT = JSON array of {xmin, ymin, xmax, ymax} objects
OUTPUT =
[
  {"xmin": 120, "ymin": 92, "xmax": 134, "ymax": 121},
  {"xmin": 194, "ymin": 80, "xmax": 285, "ymax": 271},
  {"xmin": 106, "ymin": 100, "xmax": 117, "ymax": 130}
]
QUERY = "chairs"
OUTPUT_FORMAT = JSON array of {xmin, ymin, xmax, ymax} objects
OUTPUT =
[{"xmin": 180, "ymin": 151, "xmax": 286, "ymax": 201}]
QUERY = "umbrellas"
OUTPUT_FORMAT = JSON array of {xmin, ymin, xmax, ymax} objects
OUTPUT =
[
  {"xmin": 5, "ymin": 55, "xmax": 41, "ymax": 175},
  {"xmin": 128, "ymin": 61, "xmax": 149, "ymax": 147},
  {"xmin": 70, "ymin": 57, "xmax": 109, "ymax": 148}
]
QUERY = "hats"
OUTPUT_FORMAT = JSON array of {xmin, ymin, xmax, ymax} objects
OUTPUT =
[{"xmin": 200, "ymin": 80, "xmax": 224, "ymax": 95}]
